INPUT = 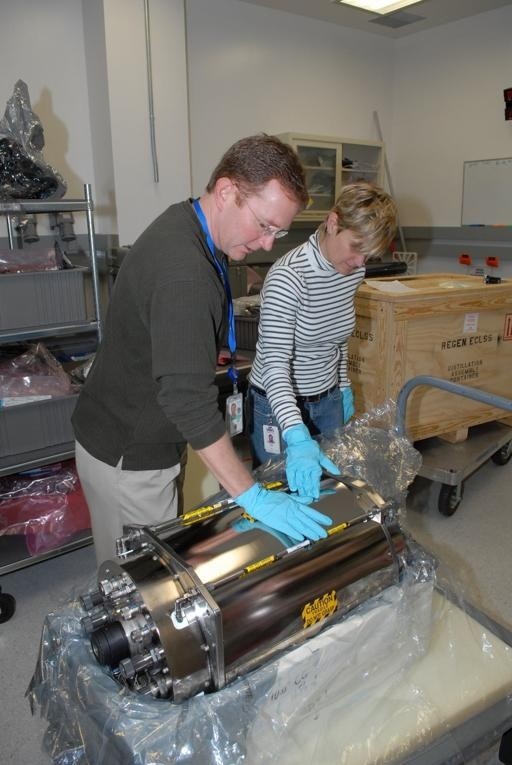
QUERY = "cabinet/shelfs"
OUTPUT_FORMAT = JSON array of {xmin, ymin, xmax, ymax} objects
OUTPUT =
[
  {"xmin": 0, "ymin": 184, "xmax": 104, "ymax": 576},
  {"xmin": 274, "ymin": 133, "xmax": 385, "ymax": 222}
]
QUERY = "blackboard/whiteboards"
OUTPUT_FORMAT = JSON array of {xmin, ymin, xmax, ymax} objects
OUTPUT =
[{"xmin": 460, "ymin": 158, "xmax": 511, "ymax": 226}]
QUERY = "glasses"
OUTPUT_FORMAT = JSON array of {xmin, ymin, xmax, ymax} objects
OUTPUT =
[{"xmin": 235, "ymin": 183, "xmax": 293, "ymax": 241}]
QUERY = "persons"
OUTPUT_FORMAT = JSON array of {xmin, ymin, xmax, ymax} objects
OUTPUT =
[
  {"xmin": 230, "ymin": 402, "xmax": 237, "ymax": 417},
  {"xmin": 267, "ymin": 435, "xmax": 275, "ymax": 443},
  {"xmin": 68, "ymin": 133, "xmax": 337, "ymax": 576},
  {"xmin": 248, "ymin": 180, "xmax": 401, "ymax": 504}
]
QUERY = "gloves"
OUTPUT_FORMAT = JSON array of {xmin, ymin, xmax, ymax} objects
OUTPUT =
[
  {"xmin": 339, "ymin": 384, "xmax": 356, "ymax": 424},
  {"xmin": 280, "ymin": 422, "xmax": 342, "ymax": 499},
  {"xmin": 235, "ymin": 482, "xmax": 333, "ymax": 545}
]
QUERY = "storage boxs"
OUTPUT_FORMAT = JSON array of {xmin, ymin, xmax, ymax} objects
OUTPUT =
[
  {"xmin": 0, "ymin": 265, "xmax": 88, "ymax": 330},
  {"xmin": 1, "ymin": 393, "xmax": 80, "ymax": 459},
  {"xmin": 346, "ymin": 274, "xmax": 512, "ymax": 444},
  {"xmin": 231, "ymin": 316, "xmax": 259, "ymax": 351}
]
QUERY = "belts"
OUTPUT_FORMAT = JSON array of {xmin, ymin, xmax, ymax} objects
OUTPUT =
[{"xmin": 248, "ymin": 381, "xmax": 338, "ymax": 404}]
